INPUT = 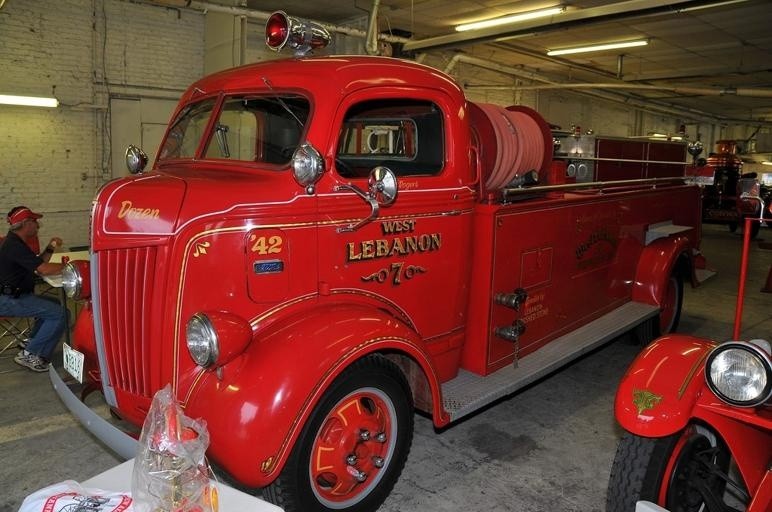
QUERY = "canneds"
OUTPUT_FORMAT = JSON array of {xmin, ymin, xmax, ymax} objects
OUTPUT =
[{"xmin": 62, "ymin": 256, "xmax": 69, "ymax": 264}]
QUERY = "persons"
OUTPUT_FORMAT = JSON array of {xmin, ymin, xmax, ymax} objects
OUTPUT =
[{"xmin": 1, "ymin": 206, "xmax": 72, "ymax": 372}]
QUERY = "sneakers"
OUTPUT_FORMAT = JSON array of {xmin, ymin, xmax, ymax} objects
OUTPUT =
[{"xmin": 14, "ymin": 338, "xmax": 51, "ymax": 371}]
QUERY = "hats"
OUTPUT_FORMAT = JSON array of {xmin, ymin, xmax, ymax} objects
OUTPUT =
[{"xmin": 7, "ymin": 206, "xmax": 43, "ymax": 225}]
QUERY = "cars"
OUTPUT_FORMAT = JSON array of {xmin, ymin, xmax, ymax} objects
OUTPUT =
[
  {"xmin": 706, "ymin": 138, "xmax": 767, "ymax": 241},
  {"xmin": 603, "ymin": 217, "xmax": 772, "ymax": 511}
]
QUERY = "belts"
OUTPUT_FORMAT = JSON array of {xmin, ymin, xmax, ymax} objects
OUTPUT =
[{"xmin": 1, "ymin": 286, "xmax": 31, "ymax": 296}]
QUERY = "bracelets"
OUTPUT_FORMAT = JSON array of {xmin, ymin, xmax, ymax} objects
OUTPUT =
[{"xmin": 46, "ymin": 246, "xmax": 54, "ymax": 253}]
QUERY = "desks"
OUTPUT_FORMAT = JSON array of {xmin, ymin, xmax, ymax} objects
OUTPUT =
[{"xmin": 34, "ymin": 250, "xmax": 91, "ymax": 346}]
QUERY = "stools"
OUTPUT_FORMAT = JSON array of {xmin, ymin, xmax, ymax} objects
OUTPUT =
[{"xmin": 1, "ymin": 316, "xmax": 40, "ymax": 354}]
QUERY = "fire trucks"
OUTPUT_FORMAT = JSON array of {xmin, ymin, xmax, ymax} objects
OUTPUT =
[{"xmin": 45, "ymin": 10, "xmax": 719, "ymax": 511}]
